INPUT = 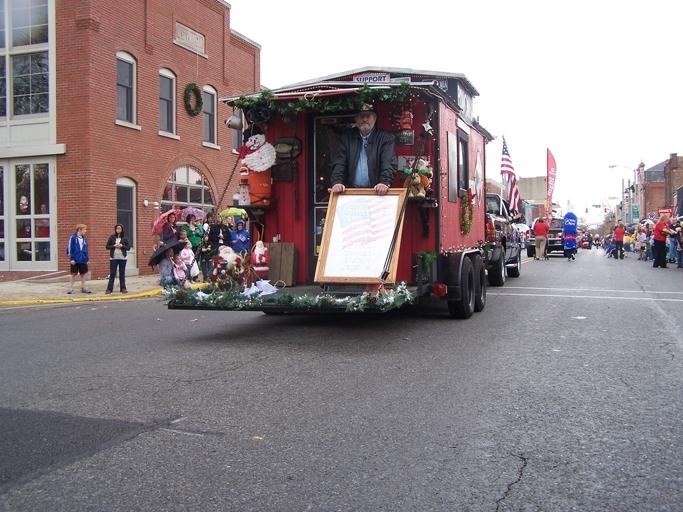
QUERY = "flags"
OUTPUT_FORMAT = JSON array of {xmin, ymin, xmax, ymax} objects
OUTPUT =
[
  {"xmin": 547, "ymin": 150, "xmax": 556, "ymax": 202},
  {"xmin": 501, "ymin": 137, "xmax": 520, "ymax": 213}
]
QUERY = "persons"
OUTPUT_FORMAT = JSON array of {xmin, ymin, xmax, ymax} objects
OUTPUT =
[
  {"xmin": 330, "ymin": 110, "xmax": 396, "ymax": 195},
  {"xmin": 533, "ymin": 215, "xmax": 683, "ymax": 268},
  {"xmin": 36, "ymin": 202, "xmax": 49, "ymax": 260},
  {"xmin": 106, "ymin": 224, "xmax": 130, "ymax": 294},
  {"xmin": 67, "ymin": 224, "xmax": 91, "ymax": 293},
  {"xmin": 159, "ymin": 213, "xmax": 250, "ymax": 289}
]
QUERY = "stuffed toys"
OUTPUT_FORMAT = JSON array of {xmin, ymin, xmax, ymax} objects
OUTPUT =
[{"xmin": 403, "ymin": 155, "xmax": 434, "ymax": 197}]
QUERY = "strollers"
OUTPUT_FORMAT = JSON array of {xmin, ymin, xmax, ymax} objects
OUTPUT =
[{"xmin": 533, "ymin": 236, "xmax": 550, "ymax": 261}]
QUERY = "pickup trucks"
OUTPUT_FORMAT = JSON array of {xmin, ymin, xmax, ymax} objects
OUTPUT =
[
  {"xmin": 485, "ymin": 193, "xmax": 523, "ymax": 285},
  {"xmin": 525, "ymin": 217, "xmax": 565, "ymax": 257}
]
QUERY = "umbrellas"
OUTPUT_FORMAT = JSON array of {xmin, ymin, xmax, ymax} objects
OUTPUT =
[
  {"xmin": 147, "ymin": 239, "xmax": 188, "ymax": 268},
  {"xmin": 180, "ymin": 206, "xmax": 206, "ymax": 222},
  {"xmin": 219, "ymin": 208, "xmax": 247, "ymax": 217},
  {"xmin": 151, "ymin": 209, "xmax": 184, "ymax": 235}
]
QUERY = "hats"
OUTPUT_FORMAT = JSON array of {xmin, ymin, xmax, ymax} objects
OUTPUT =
[{"xmin": 358, "ymin": 103, "xmax": 377, "ymax": 113}]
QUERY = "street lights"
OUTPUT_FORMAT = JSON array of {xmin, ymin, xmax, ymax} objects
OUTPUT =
[{"xmin": 608, "ymin": 163, "xmax": 637, "ymax": 222}]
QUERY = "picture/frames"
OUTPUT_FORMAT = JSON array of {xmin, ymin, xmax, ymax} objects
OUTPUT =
[{"xmin": 313, "ymin": 188, "xmax": 408, "ymax": 283}]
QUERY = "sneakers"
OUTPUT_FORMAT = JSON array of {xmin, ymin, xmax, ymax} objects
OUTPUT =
[
  {"xmin": 638, "ymin": 256, "xmax": 682, "ymax": 269},
  {"xmin": 105, "ymin": 289, "xmax": 127, "ymax": 294},
  {"xmin": 568, "ymin": 253, "xmax": 576, "ymax": 261},
  {"xmin": 67, "ymin": 287, "xmax": 92, "ymax": 294}
]
